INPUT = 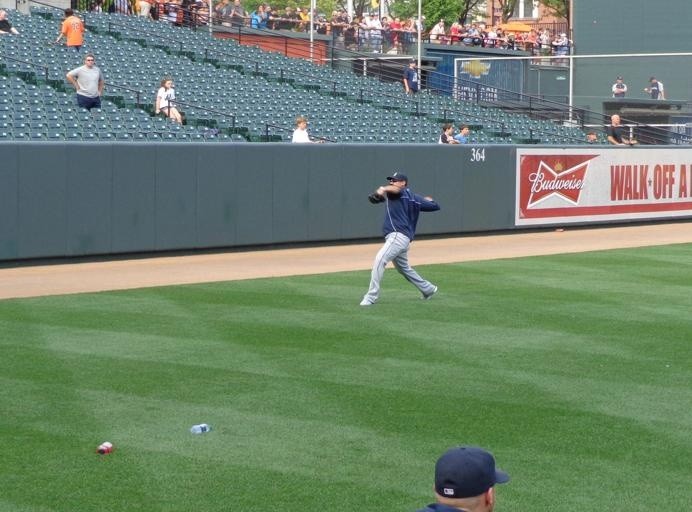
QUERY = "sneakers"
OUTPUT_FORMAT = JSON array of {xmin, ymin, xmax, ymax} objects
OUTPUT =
[
  {"xmin": 360, "ymin": 299, "xmax": 373, "ymax": 306},
  {"xmin": 421, "ymin": 286, "xmax": 437, "ymax": 299}
]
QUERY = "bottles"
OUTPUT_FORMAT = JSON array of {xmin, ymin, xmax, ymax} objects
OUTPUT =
[{"xmin": 190, "ymin": 421, "xmax": 210, "ymax": 433}]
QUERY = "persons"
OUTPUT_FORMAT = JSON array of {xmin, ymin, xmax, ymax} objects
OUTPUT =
[
  {"xmin": 187, "ymin": 1, "xmax": 571, "ymax": 66},
  {"xmin": 607, "ymin": 114, "xmax": 638, "ymax": 145},
  {"xmin": 413, "ymin": 447, "xmax": 510, "ymax": 512},
  {"xmin": 645, "ymin": 76, "xmax": 664, "ymax": 100},
  {"xmin": 612, "ymin": 75, "xmax": 628, "ymax": 97},
  {"xmin": 292, "ymin": 116, "xmax": 326, "ymax": 143},
  {"xmin": 359, "ymin": 172, "xmax": 440, "ymax": 306},
  {"xmin": 56, "ymin": 8, "xmax": 84, "ymax": 52},
  {"xmin": 438, "ymin": 123, "xmax": 455, "ymax": 144},
  {"xmin": 155, "ymin": 79, "xmax": 183, "ymax": 125},
  {"xmin": 65, "ymin": 53, "xmax": 104, "ymax": 110},
  {"xmin": 453, "ymin": 124, "xmax": 470, "ymax": 144},
  {"xmin": 0, "ymin": 8, "xmax": 21, "ymax": 35},
  {"xmin": 403, "ymin": 58, "xmax": 418, "ymax": 95}
]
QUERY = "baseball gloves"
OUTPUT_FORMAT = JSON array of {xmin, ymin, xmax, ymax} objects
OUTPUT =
[{"xmin": 368, "ymin": 191, "xmax": 385, "ymax": 204}]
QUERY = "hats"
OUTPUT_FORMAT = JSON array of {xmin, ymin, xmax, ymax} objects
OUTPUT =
[
  {"xmin": 367, "ymin": 11, "xmax": 379, "ymax": 17},
  {"xmin": 617, "ymin": 75, "xmax": 657, "ymax": 82},
  {"xmin": 432, "ymin": 445, "xmax": 512, "ymax": 499},
  {"xmin": 408, "ymin": 59, "xmax": 417, "ymax": 64},
  {"xmin": 387, "ymin": 172, "xmax": 408, "ymax": 183}
]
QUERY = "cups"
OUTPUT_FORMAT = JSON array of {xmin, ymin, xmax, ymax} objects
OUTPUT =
[{"xmin": 96, "ymin": 441, "xmax": 114, "ymax": 454}]
